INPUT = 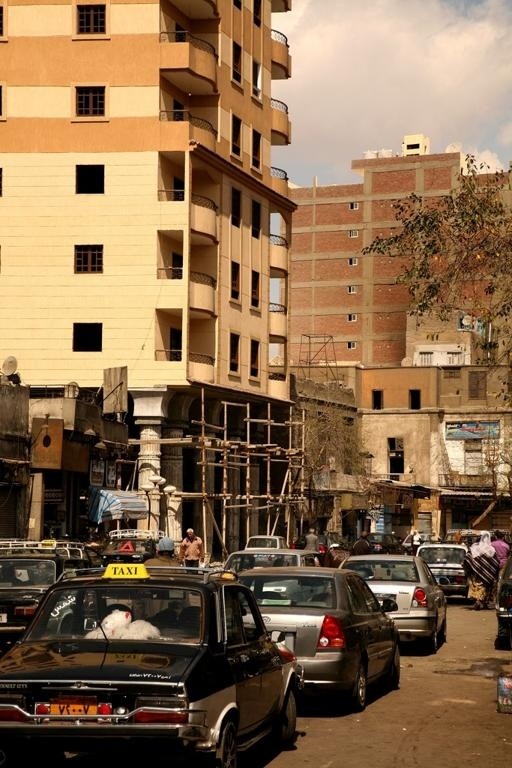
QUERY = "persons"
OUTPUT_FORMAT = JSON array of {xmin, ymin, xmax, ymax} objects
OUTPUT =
[
  {"xmin": 429, "ymin": 529, "xmax": 441, "ymax": 545},
  {"xmin": 410, "ymin": 529, "xmax": 421, "ymax": 555},
  {"xmin": 181, "ymin": 527, "xmax": 206, "ymax": 575},
  {"xmin": 144, "ymin": 535, "xmax": 188, "ymax": 573},
  {"xmin": 353, "ymin": 530, "xmax": 375, "ymax": 554},
  {"xmin": 461, "ymin": 530, "xmax": 502, "ymax": 609},
  {"xmin": 485, "ymin": 528, "xmax": 511, "ymax": 608},
  {"xmin": 303, "ymin": 526, "xmax": 320, "ymax": 552}
]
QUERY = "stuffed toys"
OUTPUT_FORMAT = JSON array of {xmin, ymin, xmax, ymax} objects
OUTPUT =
[{"xmin": 82, "ymin": 610, "xmax": 163, "ymax": 644}]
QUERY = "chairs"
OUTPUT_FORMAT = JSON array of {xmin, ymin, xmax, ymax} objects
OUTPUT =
[
  {"xmin": 257, "ymin": 590, "xmax": 328, "ymax": 603},
  {"xmin": 101, "ymin": 602, "xmax": 200, "ymax": 634},
  {"xmin": 356, "ymin": 567, "xmax": 409, "ymax": 580},
  {"xmin": 238, "ymin": 556, "xmax": 289, "ymax": 568}
]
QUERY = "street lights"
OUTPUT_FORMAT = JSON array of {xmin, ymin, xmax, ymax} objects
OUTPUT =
[
  {"xmin": 157, "ymin": 475, "xmax": 177, "ymax": 538},
  {"xmin": 140, "ymin": 473, "xmax": 161, "ymax": 531}
]
